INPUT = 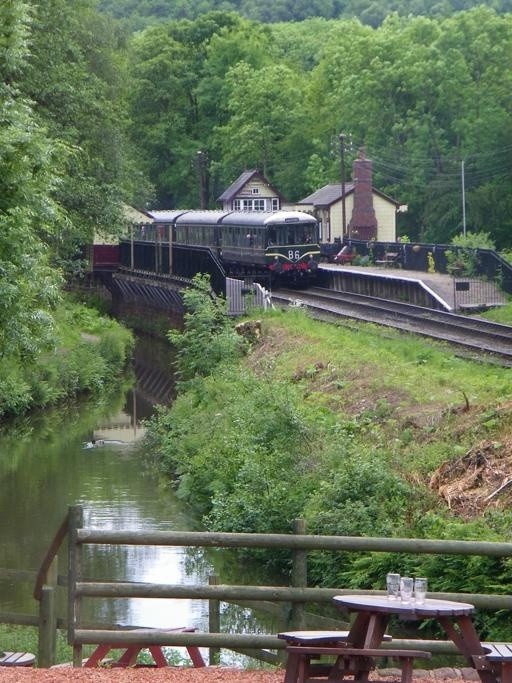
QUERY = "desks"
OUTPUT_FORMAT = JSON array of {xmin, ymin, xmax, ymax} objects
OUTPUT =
[{"xmin": 327, "ymin": 594, "xmax": 499, "ymax": 683}]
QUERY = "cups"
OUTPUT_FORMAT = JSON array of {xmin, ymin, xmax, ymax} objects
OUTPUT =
[{"xmin": 386, "ymin": 574, "xmax": 428, "ymax": 605}]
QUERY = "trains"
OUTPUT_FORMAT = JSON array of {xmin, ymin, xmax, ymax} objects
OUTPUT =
[{"xmin": 128, "ymin": 208, "xmax": 321, "ymax": 292}]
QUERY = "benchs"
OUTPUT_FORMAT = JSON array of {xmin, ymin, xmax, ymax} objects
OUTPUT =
[
  {"xmin": 481, "ymin": 644, "xmax": 512, "ymax": 683},
  {"xmin": 278, "ymin": 629, "xmax": 392, "ymax": 683},
  {"xmin": 286, "ymin": 646, "xmax": 426, "ymax": 683}
]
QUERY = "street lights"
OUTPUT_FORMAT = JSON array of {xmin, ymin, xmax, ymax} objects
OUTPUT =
[{"xmin": 337, "ymin": 133, "xmax": 347, "ymax": 240}]
